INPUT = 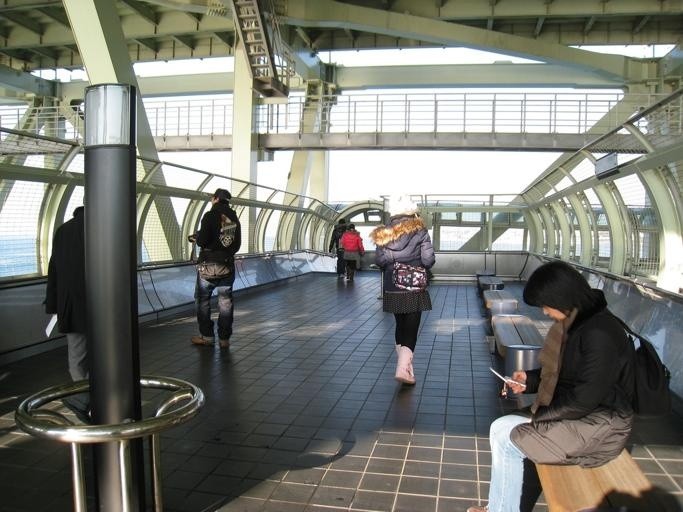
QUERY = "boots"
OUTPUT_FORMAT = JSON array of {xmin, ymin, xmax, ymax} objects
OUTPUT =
[{"xmin": 395, "ymin": 345, "xmax": 416, "ymax": 385}]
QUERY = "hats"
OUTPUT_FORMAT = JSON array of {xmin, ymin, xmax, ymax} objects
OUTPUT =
[
  {"xmin": 211, "ymin": 187, "xmax": 232, "ymax": 200},
  {"xmin": 387, "ymin": 194, "xmax": 421, "ymax": 216}
]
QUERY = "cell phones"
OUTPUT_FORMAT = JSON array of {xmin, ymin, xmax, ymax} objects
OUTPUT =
[{"xmin": 488, "ymin": 366, "xmax": 528, "ymax": 388}]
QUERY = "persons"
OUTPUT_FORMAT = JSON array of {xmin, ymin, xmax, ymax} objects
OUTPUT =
[
  {"xmin": 44, "ymin": 199, "xmax": 90, "ymax": 381},
  {"xmin": 341, "ymin": 224, "xmax": 364, "ymax": 280},
  {"xmin": 191, "ymin": 190, "xmax": 241, "ymax": 349},
  {"xmin": 373, "ymin": 196, "xmax": 434, "ymax": 384},
  {"xmin": 464, "ymin": 262, "xmax": 637, "ymax": 512},
  {"xmin": 330, "ymin": 219, "xmax": 346, "ymax": 279}
]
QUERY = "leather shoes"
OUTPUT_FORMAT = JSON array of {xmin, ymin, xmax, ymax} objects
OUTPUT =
[{"xmin": 466, "ymin": 505, "xmax": 488, "ymax": 512}]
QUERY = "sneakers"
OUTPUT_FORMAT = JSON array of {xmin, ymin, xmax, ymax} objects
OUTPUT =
[
  {"xmin": 191, "ymin": 335, "xmax": 214, "ymax": 343},
  {"xmin": 218, "ymin": 340, "xmax": 230, "ymax": 348}
]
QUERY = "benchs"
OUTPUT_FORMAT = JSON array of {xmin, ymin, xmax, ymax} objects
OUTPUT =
[
  {"xmin": 535, "ymin": 448, "xmax": 658, "ymax": 512},
  {"xmin": 481, "ymin": 289, "xmax": 519, "ymax": 317},
  {"xmin": 477, "ymin": 274, "xmax": 504, "ymax": 297},
  {"xmin": 492, "ymin": 314, "xmax": 545, "ymax": 360}
]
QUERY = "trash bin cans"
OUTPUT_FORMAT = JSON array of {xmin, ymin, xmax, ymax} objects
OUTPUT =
[{"xmin": 504, "ymin": 344, "xmax": 543, "ymax": 399}]
